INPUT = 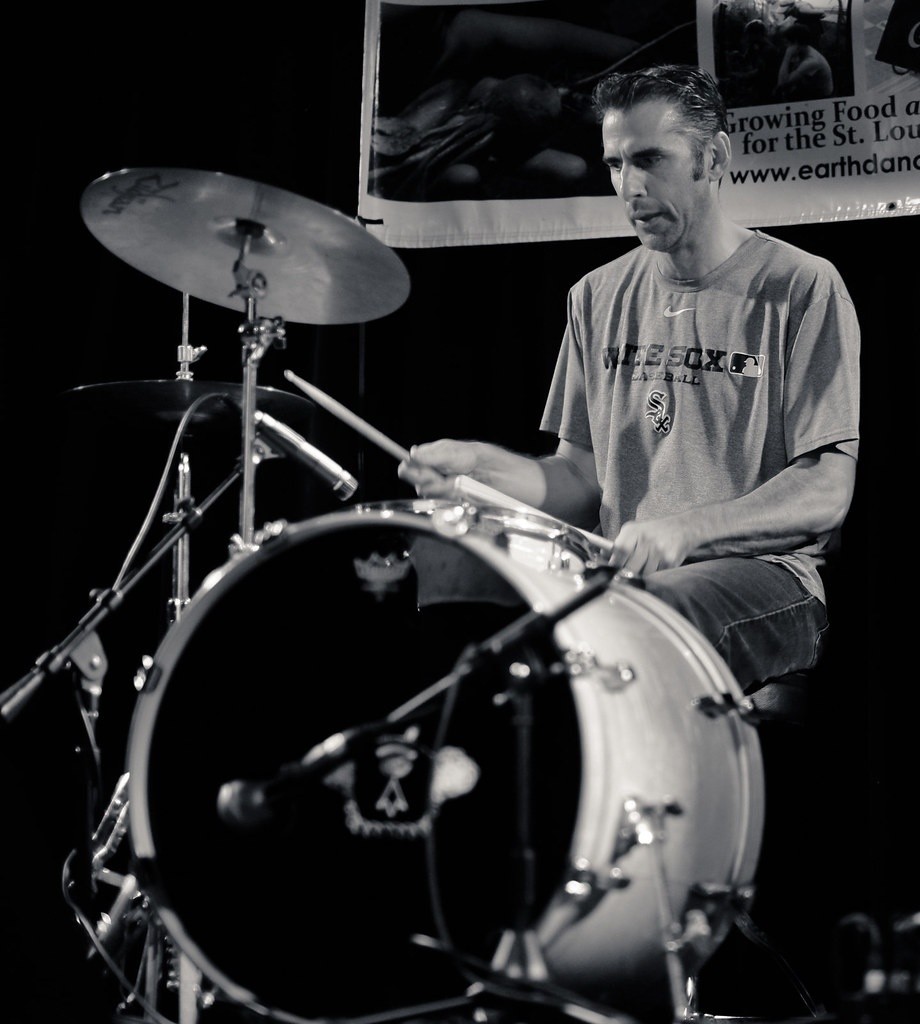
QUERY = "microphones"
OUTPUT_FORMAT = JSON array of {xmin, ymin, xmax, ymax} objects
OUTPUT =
[
  {"xmin": 214, "ymin": 728, "xmax": 396, "ymax": 828},
  {"xmin": 224, "ymin": 390, "xmax": 360, "ymax": 503}
]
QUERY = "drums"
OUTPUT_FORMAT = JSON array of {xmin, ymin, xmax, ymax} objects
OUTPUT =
[{"xmin": 124, "ymin": 503, "xmax": 767, "ymax": 1024}]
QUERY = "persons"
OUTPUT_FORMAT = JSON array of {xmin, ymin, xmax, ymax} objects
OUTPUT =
[
  {"xmin": 397, "ymin": 65, "xmax": 860, "ymax": 698},
  {"xmin": 713, "ymin": 1, "xmax": 853, "ymax": 108},
  {"xmin": 376, "ymin": 74, "xmax": 599, "ymax": 203}
]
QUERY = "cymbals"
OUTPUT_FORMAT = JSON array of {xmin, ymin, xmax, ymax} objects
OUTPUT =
[
  {"xmin": 59, "ymin": 380, "xmax": 316, "ymax": 448},
  {"xmin": 80, "ymin": 166, "xmax": 411, "ymax": 326}
]
